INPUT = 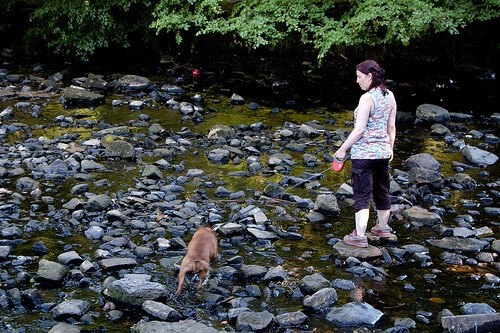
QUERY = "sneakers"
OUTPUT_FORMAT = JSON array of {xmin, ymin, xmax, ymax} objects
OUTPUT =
[
  {"xmin": 343, "ymin": 229, "xmax": 369, "ymax": 247},
  {"xmin": 371, "ymin": 219, "xmax": 391, "ymax": 237}
]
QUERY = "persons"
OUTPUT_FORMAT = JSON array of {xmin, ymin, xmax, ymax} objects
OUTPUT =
[{"xmin": 334, "ymin": 60, "xmax": 397, "ymax": 247}]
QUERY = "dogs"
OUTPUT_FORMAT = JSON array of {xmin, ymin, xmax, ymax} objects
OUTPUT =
[{"xmin": 175, "ymin": 227, "xmax": 218, "ymax": 296}]
